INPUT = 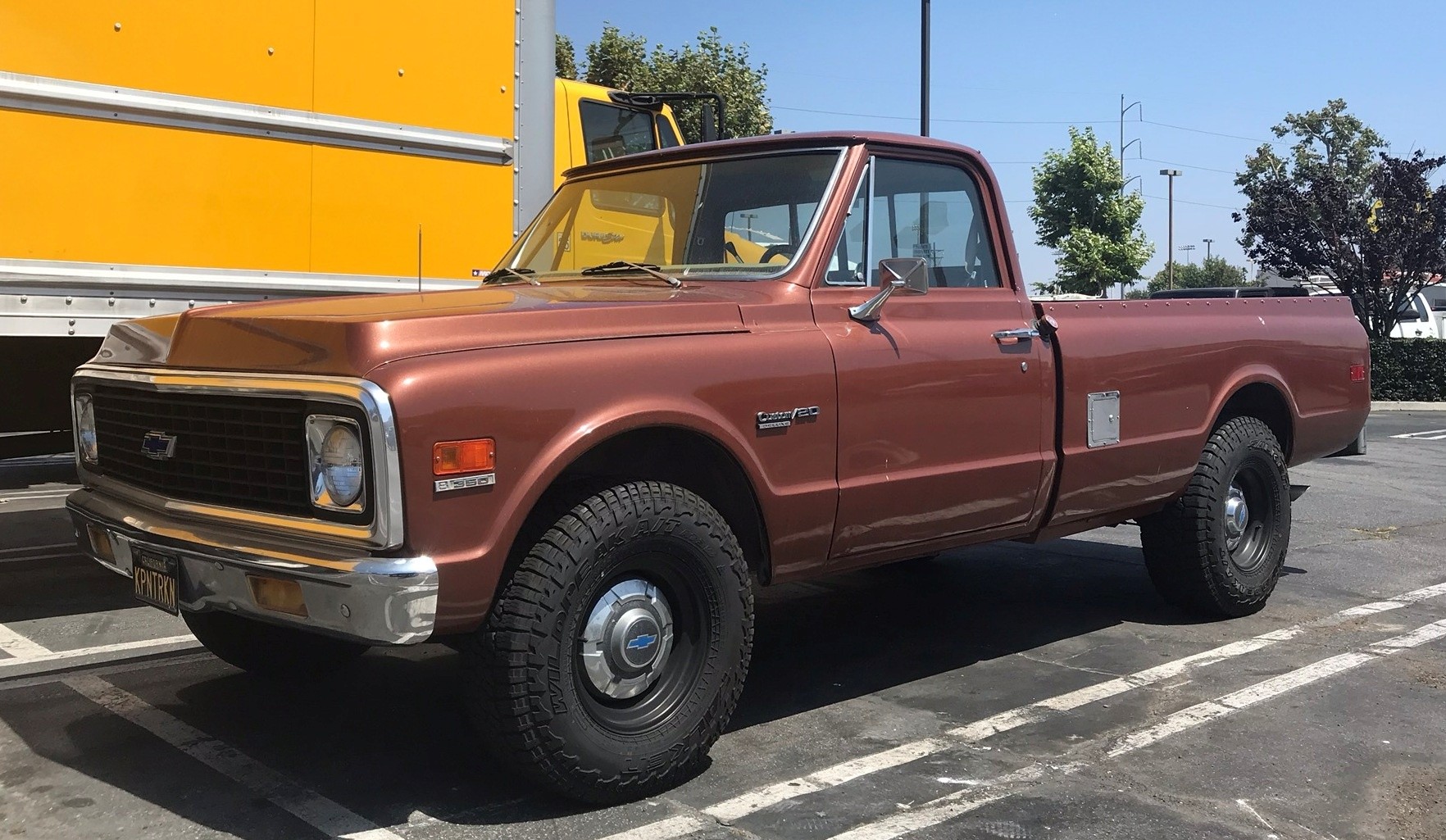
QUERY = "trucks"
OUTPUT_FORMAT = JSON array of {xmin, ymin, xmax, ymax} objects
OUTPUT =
[{"xmin": 0, "ymin": 0, "xmax": 805, "ymax": 492}]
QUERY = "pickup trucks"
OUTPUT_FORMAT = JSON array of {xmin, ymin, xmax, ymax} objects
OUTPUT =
[{"xmin": 68, "ymin": 129, "xmax": 1372, "ymax": 811}]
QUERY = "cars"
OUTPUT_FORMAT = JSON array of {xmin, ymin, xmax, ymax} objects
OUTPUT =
[{"xmin": 1302, "ymin": 271, "xmax": 1446, "ymax": 341}]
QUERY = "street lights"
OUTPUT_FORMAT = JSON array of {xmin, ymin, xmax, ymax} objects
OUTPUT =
[
  {"xmin": 1203, "ymin": 239, "xmax": 1215, "ymax": 262},
  {"xmin": 1179, "ymin": 244, "xmax": 1196, "ymax": 266},
  {"xmin": 1159, "ymin": 168, "xmax": 1182, "ymax": 290}
]
